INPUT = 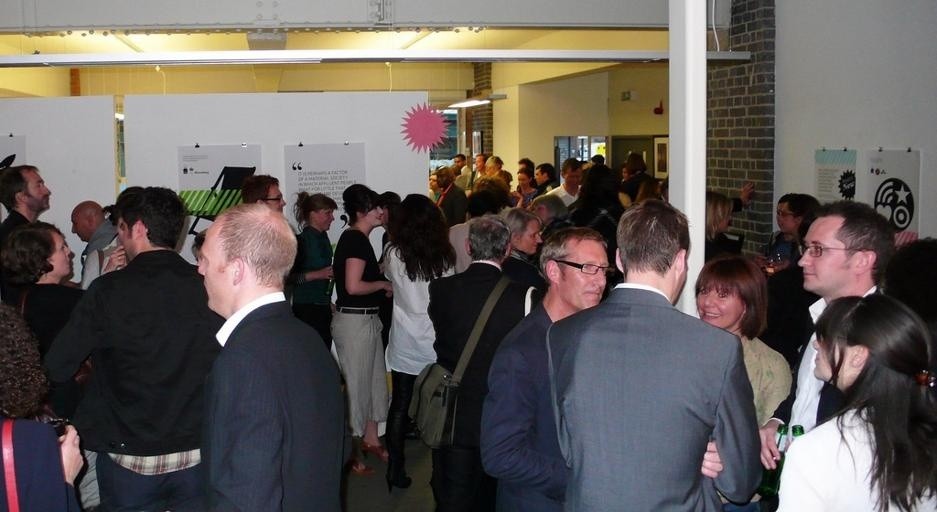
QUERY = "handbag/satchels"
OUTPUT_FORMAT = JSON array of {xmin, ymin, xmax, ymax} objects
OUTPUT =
[{"xmin": 407, "ymin": 363, "xmax": 460, "ymax": 450}]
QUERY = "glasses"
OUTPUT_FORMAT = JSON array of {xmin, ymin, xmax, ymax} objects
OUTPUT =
[
  {"xmin": 798, "ymin": 244, "xmax": 860, "ymax": 257},
  {"xmin": 777, "ymin": 209, "xmax": 793, "ymax": 217},
  {"xmin": 261, "ymin": 194, "xmax": 283, "ymax": 201},
  {"xmin": 545, "ymin": 258, "xmax": 615, "ymax": 277}
]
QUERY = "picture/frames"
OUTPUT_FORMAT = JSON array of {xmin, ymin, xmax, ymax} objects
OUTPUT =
[
  {"xmin": 472, "ymin": 131, "xmax": 483, "ymax": 159},
  {"xmin": 652, "ymin": 135, "xmax": 669, "ymax": 180}
]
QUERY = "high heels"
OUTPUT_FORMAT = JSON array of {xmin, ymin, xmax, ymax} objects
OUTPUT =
[{"xmin": 383, "ymin": 465, "xmax": 411, "ymax": 493}]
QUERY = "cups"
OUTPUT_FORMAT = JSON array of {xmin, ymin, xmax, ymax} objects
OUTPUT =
[
  {"xmin": 47, "ymin": 419, "xmax": 66, "ymax": 444},
  {"xmin": 763, "ymin": 252, "xmax": 784, "ymax": 274}
]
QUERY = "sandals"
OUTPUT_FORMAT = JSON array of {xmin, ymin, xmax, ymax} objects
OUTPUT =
[
  {"xmin": 359, "ymin": 436, "xmax": 388, "ymax": 459},
  {"xmin": 346, "ymin": 451, "xmax": 374, "ymax": 474}
]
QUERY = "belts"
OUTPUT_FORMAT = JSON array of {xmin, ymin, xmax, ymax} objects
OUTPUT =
[{"xmin": 337, "ymin": 307, "xmax": 379, "ymax": 314}]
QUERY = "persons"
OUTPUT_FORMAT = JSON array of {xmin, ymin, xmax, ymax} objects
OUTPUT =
[{"xmin": 1, "ymin": 150, "xmax": 937, "ymax": 512}]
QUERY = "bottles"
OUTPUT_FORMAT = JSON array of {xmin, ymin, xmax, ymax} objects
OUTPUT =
[
  {"xmin": 322, "ymin": 256, "xmax": 335, "ymax": 297},
  {"xmin": 790, "ymin": 424, "xmax": 804, "ymax": 439},
  {"xmin": 757, "ymin": 424, "xmax": 788, "ymax": 497}
]
